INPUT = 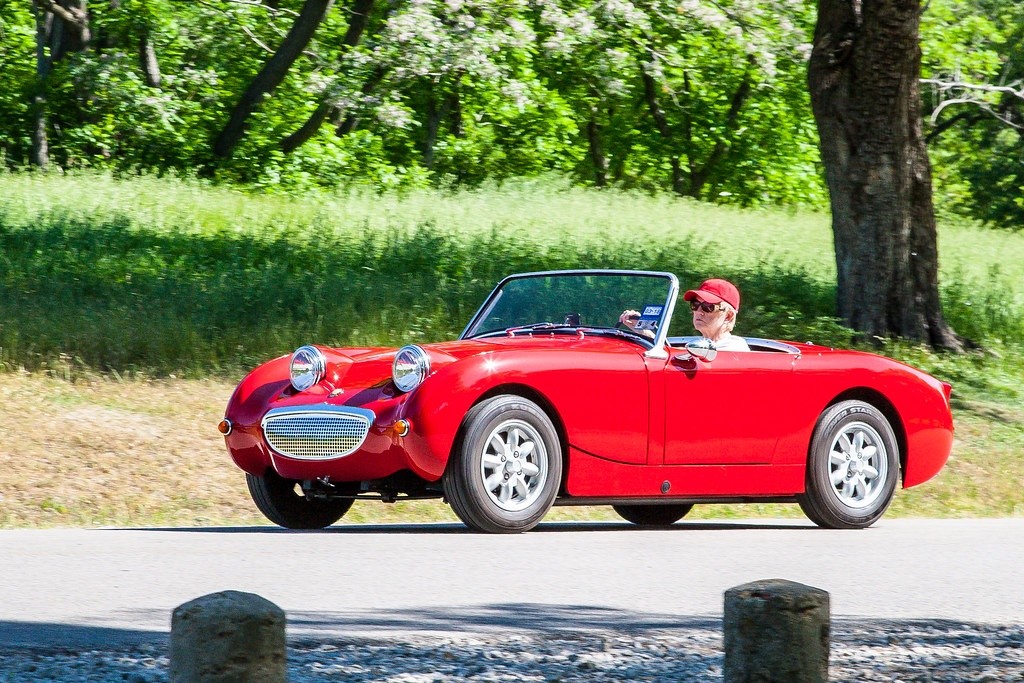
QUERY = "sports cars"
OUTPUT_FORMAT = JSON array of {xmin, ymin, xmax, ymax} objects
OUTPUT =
[{"xmin": 215, "ymin": 270, "xmax": 955, "ymax": 530}]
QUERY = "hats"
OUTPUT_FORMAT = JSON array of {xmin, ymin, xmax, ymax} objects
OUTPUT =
[{"xmin": 684, "ymin": 278, "xmax": 740, "ymax": 311}]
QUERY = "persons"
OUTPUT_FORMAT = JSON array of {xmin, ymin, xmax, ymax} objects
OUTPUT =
[{"xmin": 619, "ymin": 278, "xmax": 754, "ymax": 352}]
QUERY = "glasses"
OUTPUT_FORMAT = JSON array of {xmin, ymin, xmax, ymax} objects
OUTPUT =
[{"xmin": 690, "ymin": 298, "xmax": 725, "ymax": 312}]
